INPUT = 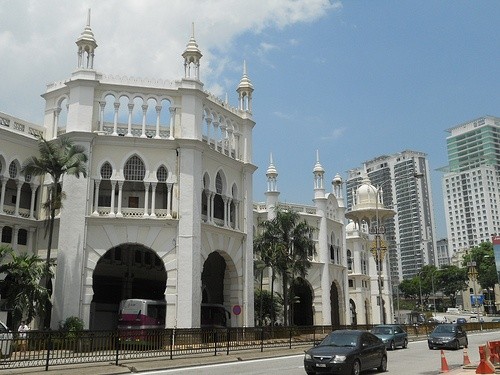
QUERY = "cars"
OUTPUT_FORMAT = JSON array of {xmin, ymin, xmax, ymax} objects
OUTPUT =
[
  {"xmin": 370, "ymin": 325, "xmax": 408, "ymax": 350},
  {"xmin": 427, "ymin": 317, "xmax": 468, "ymax": 350},
  {"xmin": 304, "ymin": 330, "xmax": 387, "ymax": 375},
  {"xmin": 0, "ymin": 322, "xmax": 13, "ymax": 359}
]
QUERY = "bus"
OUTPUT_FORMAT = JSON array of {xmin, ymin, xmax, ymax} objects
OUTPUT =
[{"xmin": 116, "ymin": 298, "xmax": 231, "ymax": 349}]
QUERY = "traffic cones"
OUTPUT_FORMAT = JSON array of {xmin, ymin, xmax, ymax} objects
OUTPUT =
[
  {"xmin": 462, "ymin": 346, "xmax": 471, "ymax": 366},
  {"xmin": 440, "ymin": 349, "xmax": 450, "ymax": 373}
]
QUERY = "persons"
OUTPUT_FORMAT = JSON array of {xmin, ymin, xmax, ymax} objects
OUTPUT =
[
  {"xmin": 265, "ymin": 321, "xmax": 282, "ymax": 326},
  {"xmin": 481, "ymin": 318, "xmax": 484, "ymax": 330},
  {"xmin": 18, "ymin": 321, "xmax": 29, "ymax": 357}
]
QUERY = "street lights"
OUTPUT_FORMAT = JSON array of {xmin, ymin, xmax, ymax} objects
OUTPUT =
[
  {"xmin": 336, "ymin": 172, "xmax": 425, "ymax": 325},
  {"xmin": 419, "ymin": 268, "xmax": 448, "ymax": 317}
]
